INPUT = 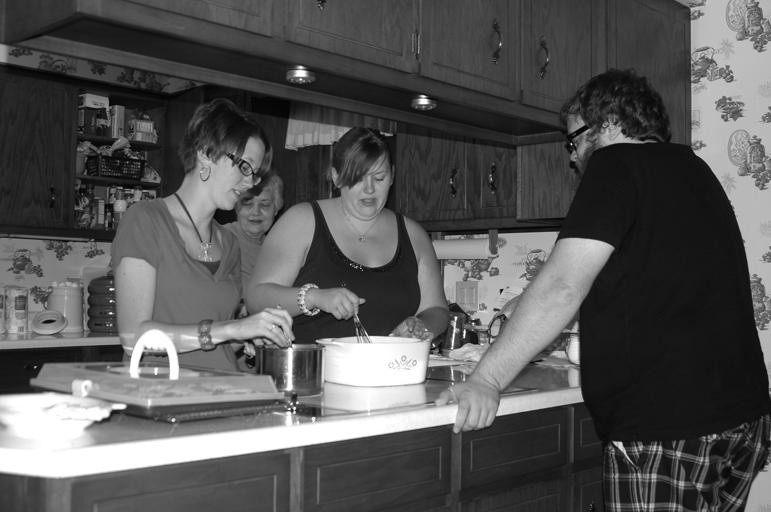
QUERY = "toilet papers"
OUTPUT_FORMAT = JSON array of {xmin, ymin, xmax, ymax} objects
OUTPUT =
[{"xmin": 432, "ymin": 238, "xmax": 500, "ymax": 259}]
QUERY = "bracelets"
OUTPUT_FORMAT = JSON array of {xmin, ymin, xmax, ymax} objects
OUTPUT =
[
  {"xmin": 297, "ymin": 282, "xmax": 321, "ymax": 318},
  {"xmin": 197, "ymin": 319, "xmax": 217, "ymax": 351}
]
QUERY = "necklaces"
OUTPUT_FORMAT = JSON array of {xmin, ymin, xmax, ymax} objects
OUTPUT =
[
  {"xmin": 172, "ymin": 191, "xmax": 217, "ymax": 263},
  {"xmin": 340, "ymin": 196, "xmax": 379, "ymax": 243}
]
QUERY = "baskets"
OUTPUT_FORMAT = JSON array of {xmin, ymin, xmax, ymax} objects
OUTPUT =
[{"xmin": 86, "ymin": 153, "xmax": 146, "ymax": 180}]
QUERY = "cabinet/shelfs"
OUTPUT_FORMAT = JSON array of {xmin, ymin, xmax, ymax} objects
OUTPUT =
[
  {"xmin": 421, "ymin": 1, "xmax": 601, "ymax": 142},
  {"xmin": 1, "ymin": 356, "xmax": 609, "ymax": 511},
  {"xmin": 1, "ymin": 0, "xmax": 421, "ymax": 113},
  {"xmin": 0, "ymin": 345, "xmax": 122, "ymax": 394},
  {"xmin": 601, "ymin": 1, "xmax": 693, "ymax": 145}
]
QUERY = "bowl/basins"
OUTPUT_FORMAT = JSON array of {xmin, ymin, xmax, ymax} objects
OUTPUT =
[
  {"xmin": 315, "ymin": 335, "xmax": 432, "ymax": 387},
  {"xmin": 0, "ymin": 392, "xmax": 127, "ymax": 439}
]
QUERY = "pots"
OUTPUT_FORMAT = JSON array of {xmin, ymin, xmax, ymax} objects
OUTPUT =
[{"xmin": 255, "ymin": 344, "xmax": 326, "ymax": 396}]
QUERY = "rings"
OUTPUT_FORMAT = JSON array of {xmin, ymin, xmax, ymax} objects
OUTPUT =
[
  {"xmin": 424, "ymin": 326, "xmax": 431, "ymax": 332},
  {"xmin": 268, "ymin": 322, "xmax": 277, "ymax": 332}
]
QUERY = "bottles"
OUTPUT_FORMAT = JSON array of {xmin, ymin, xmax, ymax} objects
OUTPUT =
[
  {"xmin": 445, "ymin": 312, "xmax": 465, "ymax": 350},
  {"xmin": 113, "ymin": 189, "xmax": 127, "ymax": 230},
  {"xmin": 4, "ymin": 287, "xmax": 28, "ymax": 335}
]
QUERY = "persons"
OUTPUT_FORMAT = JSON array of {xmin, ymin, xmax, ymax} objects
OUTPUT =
[
  {"xmin": 221, "ymin": 170, "xmax": 285, "ymax": 299},
  {"xmin": 242, "ymin": 125, "xmax": 451, "ymax": 341},
  {"xmin": 108, "ymin": 98, "xmax": 296, "ymax": 374},
  {"xmin": 434, "ymin": 67, "xmax": 771, "ymax": 512}
]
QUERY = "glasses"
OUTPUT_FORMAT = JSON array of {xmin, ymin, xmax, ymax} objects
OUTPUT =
[
  {"xmin": 221, "ymin": 150, "xmax": 262, "ymax": 186},
  {"xmin": 565, "ymin": 121, "xmax": 595, "ymax": 152}
]
